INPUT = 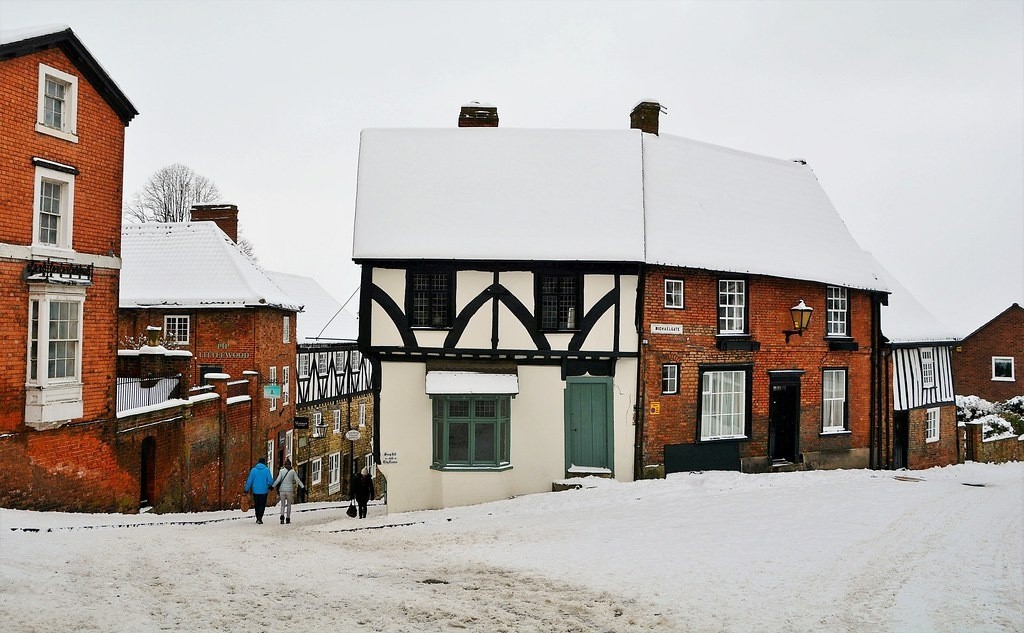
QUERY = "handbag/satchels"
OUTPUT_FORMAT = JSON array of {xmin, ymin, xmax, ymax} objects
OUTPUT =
[
  {"xmin": 276, "ymin": 479, "xmax": 281, "ymax": 491},
  {"xmin": 346, "ymin": 499, "xmax": 357, "ymax": 518},
  {"xmin": 241, "ymin": 493, "xmax": 249, "ymax": 512}
]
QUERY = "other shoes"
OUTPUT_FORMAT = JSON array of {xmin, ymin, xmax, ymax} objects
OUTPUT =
[
  {"xmin": 362, "ymin": 515, "xmax": 366, "ymax": 518},
  {"xmin": 256, "ymin": 518, "xmax": 263, "ymax": 524},
  {"xmin": 359, "ymin": 516, "xmax": 362, "ymax": 518}
]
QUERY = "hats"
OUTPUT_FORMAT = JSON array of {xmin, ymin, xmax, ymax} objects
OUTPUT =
[
  {"xmin": 284, "ymin": 459, "xmax": 291, "ymax": 466},
  {"xmin": 361, "ymin": 466, "xmax": 368, "ymax": 476},
  {"xmin": 258, "ymin": 458, "xmax": 265, "ymax": 464}
]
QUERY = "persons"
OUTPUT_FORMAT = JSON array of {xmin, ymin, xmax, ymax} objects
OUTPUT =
[
  {"xmin": 269, "ymin": 457, "xmax": 308, "ymax": 525},
  {"xmin": 243, "ymin": 458, "xmax": 274, "ymax": 524},
  {"xmin": 352, "ymin": 466, "xmax": 375, "ymax": 519}
]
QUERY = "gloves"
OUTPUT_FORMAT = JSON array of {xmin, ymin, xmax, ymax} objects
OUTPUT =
[
  {"xmin": 349, "ymin": 495, "xmax": 354, "ymax": 500},
  {"xmin": 371, "ymin": 496, "xmax": 375, "ymax": 501},
  {"xmin": 268, "ymin": 485, "xmax": 274, "ymax": 491},
  {"xmin": 244, "ymin": 490, "xmax": 249, "ymax": 492}
]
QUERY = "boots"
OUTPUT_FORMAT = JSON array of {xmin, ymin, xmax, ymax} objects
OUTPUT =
[
  {"xmin": 286, "ymin": 517, "xmax": 290, "ymax": 524},
  {"xmin": 280, "ymin": 514, "xmax": 285, "ymax": 524}
]
